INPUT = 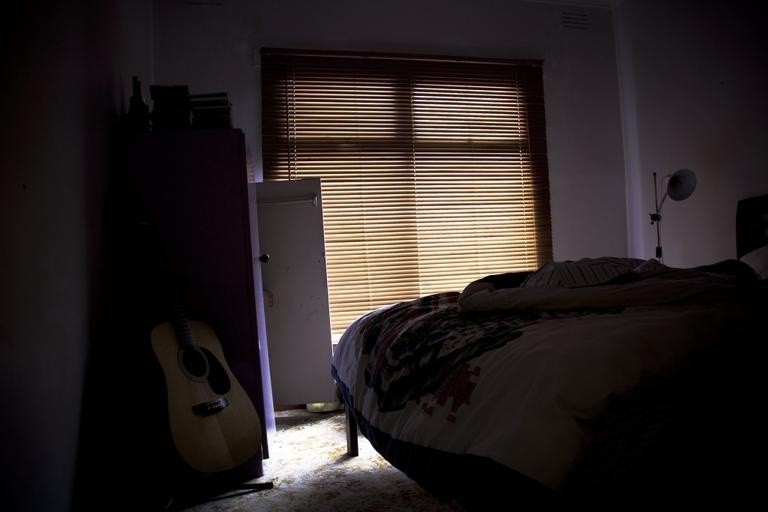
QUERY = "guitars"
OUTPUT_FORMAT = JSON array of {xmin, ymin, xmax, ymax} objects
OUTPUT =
[{"xmin": 143, "ymin": 306, "xmax": 265, "ymax": 478}]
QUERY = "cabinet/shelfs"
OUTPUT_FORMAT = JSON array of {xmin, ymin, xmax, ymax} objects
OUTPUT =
[{"xmin": 100, "ymin": 129, "xmax": 338, "ymax": 485}]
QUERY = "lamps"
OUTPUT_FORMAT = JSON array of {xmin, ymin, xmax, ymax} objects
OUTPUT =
[{"xmin": 653, "ymin": 169, "xmax": 697, "ymax": 264}]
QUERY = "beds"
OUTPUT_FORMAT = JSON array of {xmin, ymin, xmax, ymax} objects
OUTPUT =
[{"xmin": 329, "ymin": 193, "xmax": 767, "ymax": 511}]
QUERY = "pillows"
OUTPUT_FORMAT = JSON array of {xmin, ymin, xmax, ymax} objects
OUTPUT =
[{"xmin": 739, "ymin": 245, "xmax": 768, "ymax": 280}]
